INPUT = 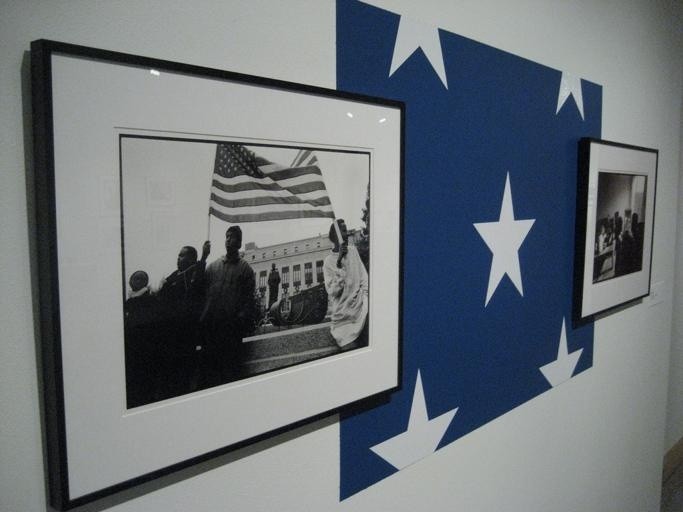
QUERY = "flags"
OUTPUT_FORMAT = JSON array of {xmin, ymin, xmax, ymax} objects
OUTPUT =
[{"xmin": 207, "ymin": 143, "xmax": 335, "ymax": 223}]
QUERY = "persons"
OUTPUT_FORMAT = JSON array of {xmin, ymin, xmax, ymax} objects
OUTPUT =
[
  {"xmin": 124, "ymin": 226, "xmax": 256, "ymax": 407},
  {"xmin": 322, "ymin": 218, "xmax": 368, "ymax": 351},
  {"xmin": 267, "ymin": 263, "xmax": 281, "ymax": 310},
  {"xmin": 601, "ymin": 210, "xmax": 644, "ymax": 274}
]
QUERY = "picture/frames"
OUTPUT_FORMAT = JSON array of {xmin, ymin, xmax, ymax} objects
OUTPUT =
[
  {"xmin": 30, "ymin": 38, "xmax": 404, "ymax": 511},
  {"xmin": 575, "ymin": 138, "xmax": 658, "ymax": 323}
]
